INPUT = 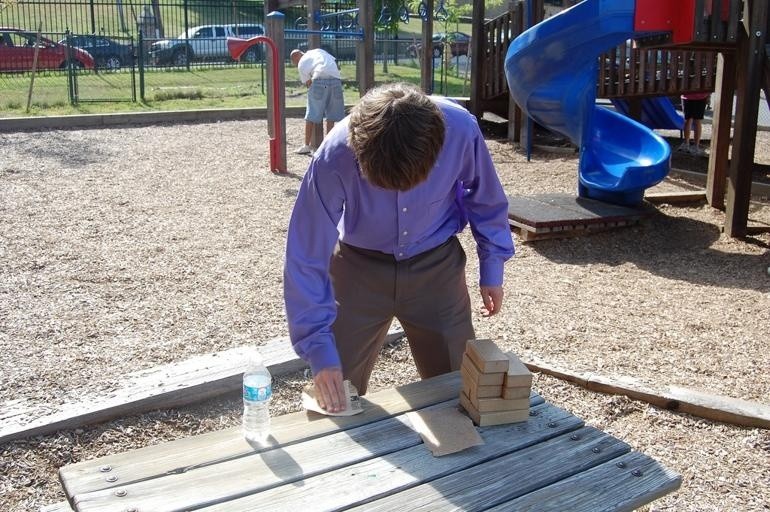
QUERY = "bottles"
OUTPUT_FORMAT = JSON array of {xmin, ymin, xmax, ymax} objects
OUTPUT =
[{"xmin": 241, "ymin": 350, "xmax": 274, "ymax": 445}]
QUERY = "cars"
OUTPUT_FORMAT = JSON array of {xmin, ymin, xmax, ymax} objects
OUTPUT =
[
  {"xmin": 0, "ymin": 27, "xmax": 95, "ymax": 74},
  {"xmin": 55, "ymin": 33, "xmax": 133, "ymax": 70},
  {"xmin": 406, "ymin": 31, "xmax": 471, "ymax": 58}
]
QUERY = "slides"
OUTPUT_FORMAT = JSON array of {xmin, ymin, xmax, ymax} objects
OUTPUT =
[{"xmin": 505, "ymin": 0, "xmax": 697, "ymax": 205}]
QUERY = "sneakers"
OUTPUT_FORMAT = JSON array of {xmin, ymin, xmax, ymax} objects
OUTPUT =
[{"xmin": 296, "ymin": 146, "xmax": 311, "ymax": 154}]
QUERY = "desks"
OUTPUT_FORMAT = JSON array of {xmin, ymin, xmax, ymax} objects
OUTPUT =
[{"xmin": 58, "ymin": 368, "xmax": 684, "ymax": 512}]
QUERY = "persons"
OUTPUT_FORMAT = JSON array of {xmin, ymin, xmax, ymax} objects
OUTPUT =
[
  {"xmin": 282, "ymin": 81, "xmax": 518, "ymax": 413},
  {"xmin": 289, "ymin": 48, "xmax": 347, "ymax": 157},
  {"xmin": 676, "ymin": 90, "xmax": 710, "ymax": 155}
]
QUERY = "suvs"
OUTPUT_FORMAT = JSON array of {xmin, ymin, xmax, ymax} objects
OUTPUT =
[
  {"xmin": 297, "ymin": 31, "xmax": 376, "ymax": 55},
  {"xmin": 148, "ymin": 23, "xmax": 265, "ymax": 67}
]
